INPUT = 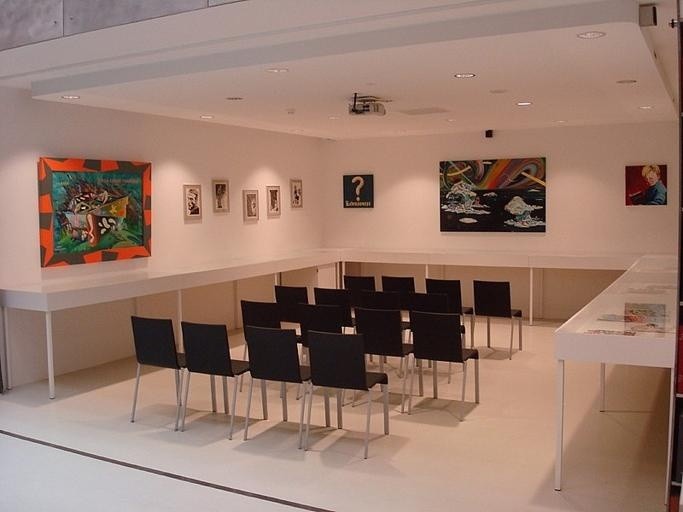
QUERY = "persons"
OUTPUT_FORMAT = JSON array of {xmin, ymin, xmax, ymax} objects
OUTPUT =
[{"xmin": 642, "ymin": 165, "xmax": 667, "ymax": 205}]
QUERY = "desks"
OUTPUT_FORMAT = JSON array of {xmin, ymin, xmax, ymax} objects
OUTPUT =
[{"xmin": 1, "ymin": 246, "xmax": 678, "ymax": 492}]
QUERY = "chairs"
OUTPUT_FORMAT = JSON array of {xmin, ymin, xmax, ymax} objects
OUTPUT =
[
  {"xmin": 243, "ymin": 327, "xmax": 331, "ymax": 450},
  {"xmin": 179, "ymin": 321, "xmax": 265, "ymax": 440},
  {"xmin": 242, "ymin": 275, "xmax": 522, "ymax": 421},
  {"xmin": 304, "ymin": 330, "xmax": 390, "ymax": 460},
  {"xmin": 131, "ymin": 316, "xmax": 217, "ymax": 431}
]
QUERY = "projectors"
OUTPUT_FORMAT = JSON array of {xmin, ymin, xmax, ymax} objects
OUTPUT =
[{"xmin": 349, "ymin": 104, "xmax": 385, "ymax": 116}]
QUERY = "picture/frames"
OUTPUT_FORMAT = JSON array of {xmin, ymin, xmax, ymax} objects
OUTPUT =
[{"xmin": 38, "ymin": 156, "xmax": 152, "ymax": 268}]
